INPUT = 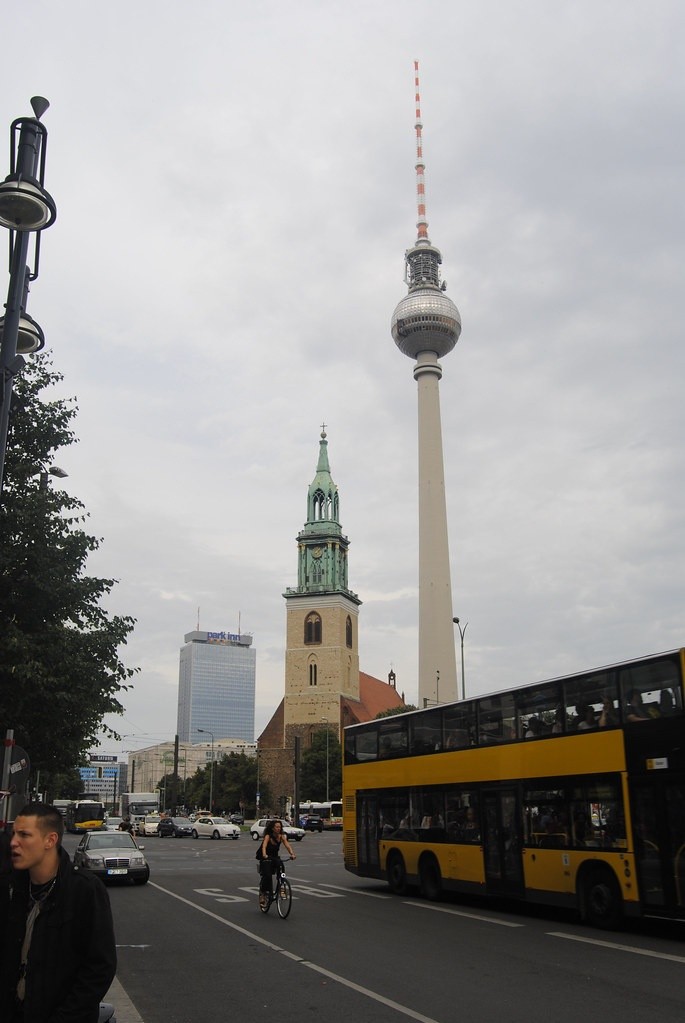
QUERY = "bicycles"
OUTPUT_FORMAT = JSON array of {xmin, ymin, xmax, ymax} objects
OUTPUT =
[{"xmin": 256, "ymin": 855, "xmax": 293, "ymax": 919}]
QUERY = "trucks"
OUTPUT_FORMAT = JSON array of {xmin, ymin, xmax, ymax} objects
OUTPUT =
[{"xmin": 119, "ymin": 792, "xmax": 160, "ymax": 833}]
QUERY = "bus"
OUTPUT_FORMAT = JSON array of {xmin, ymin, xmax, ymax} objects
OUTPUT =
[
  {"xmin": 65, "ymin": 799, "xmax": 107, "ymax": 834},
  {"xmin": 291, "ymin": 798, "xmax": 343, "ymax": 832},
  {"xmin": 340, "ymin": 645, "xmax": 685, "ymax": 935}
]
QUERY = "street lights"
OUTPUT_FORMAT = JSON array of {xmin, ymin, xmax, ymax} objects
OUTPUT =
[
  {"xmin": 153, "ymin": 753, "xmax": 167, "ymax": 811},
  {"xmin": 321, "ymin": 716, "xmax": 330, "ymax": 802},
  {"xmin": 453, "ymin": 616, "xmax": 470, "ymax": 699},
  {"xmin": 172, "ymin": 743, "xmax": 187, "ymax": 812},
  {"xmin": 196, "ymin": 728, "xmax": 214, "ymax": 812},
  {"xmin": 253, "ymin": 739, "xmax": 262, "ymax": 822},
  {"xmin": 435, "ymin": 669, "xmax": 441, "ymax": 706},
  {"xmin": 0, "ymin": 96, "xmax": 60, "ymax": 489},
  {"xmin": 143, "ymin": 759, "xmax": 155, "ymax": 793},
  {"xmin": 0, "ymin": 465, "xmax": 72, "ymax": 831}
]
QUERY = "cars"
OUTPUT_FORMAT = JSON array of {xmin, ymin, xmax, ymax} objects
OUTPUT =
[
  {"xmin": 188, "ymin": 810, "xmax": 213, "ymax": 822},
  {"xmin": 249, "ymin": 818, "xmax": 306, "ymax": 841},
  {"xmin": 190, "ymin": 816, "xmax": 241, "ymax": 840},
  {"xmin": 157, "ymin": 816, "xmax": 193, "ymax": 838},
  {"xmin": 140, "ymin": 815, "xmax": 162, "ymax": 836},
  {"xmin": 73, "ymin": 830, "xmax": 150, "ymax": 886},
  {"xmin": 227, "ymin": 814, "xmax": 245, "ymax": 825}
]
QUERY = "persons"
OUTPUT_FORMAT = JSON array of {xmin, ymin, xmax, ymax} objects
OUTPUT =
[
  {"xmin": 510, "ymin": 709, "xmax": 569, "ymax": 739},
  {"xmin": 0, "ymin": 803, "xmax": 117, "ymax": 1023},
  {"xmin": 381, "ymin": 801, "xmax": 618, "ymax": 849},
  {"xmin": 260, "ymin": 821, "xmax": 296, "ymax": 907},
  {"xmin": 573, "ymin": 696, "xmax": 618, "ymax": 730},
  {"xmin": 379, "ymin": 727, "xmax": 488, "ymax": 758},
  {"xmin": 625, "ymin": 690, "xmax": 673, "ymax": 722},
  {"xmin": 119, "ymin": 818, "xmax": 135, "ymax": 837}
]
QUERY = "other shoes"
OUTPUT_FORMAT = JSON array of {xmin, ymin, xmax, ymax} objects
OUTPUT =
[
  {"xmin": 281, "ymin": 891, "xmax": 286, "ymax": 900},
  {"xmin": 259, "ymin": 894, "xmax": 266, "ymax": 907}
]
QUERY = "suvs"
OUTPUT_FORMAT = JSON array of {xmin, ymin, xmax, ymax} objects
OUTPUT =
[{"xmin": 303, "ymin": 813, "xmax": 324, "ymax": 833}]
